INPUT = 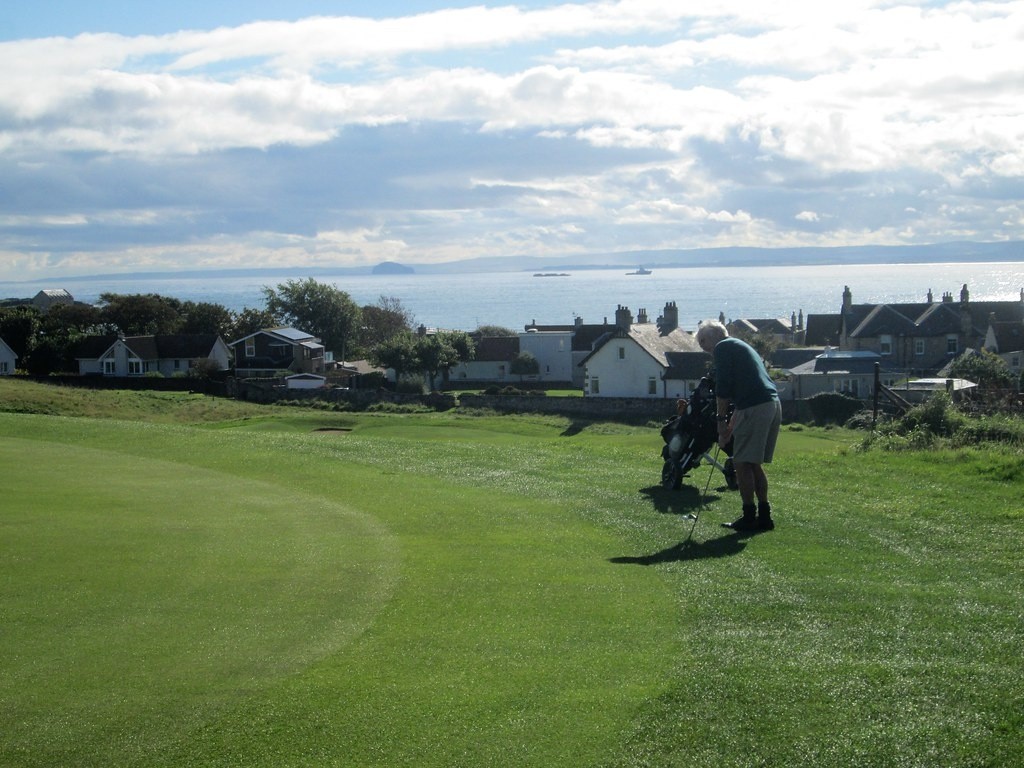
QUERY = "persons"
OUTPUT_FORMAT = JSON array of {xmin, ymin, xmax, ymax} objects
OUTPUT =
[{"xmin": 693, "ymin": 319, "xmax": 783, "ymax": 529}]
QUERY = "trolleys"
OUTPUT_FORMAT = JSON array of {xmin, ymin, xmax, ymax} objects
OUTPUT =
[{"xmin": 661, "ymin": 367, "xmax": 738, "ymax": 489}]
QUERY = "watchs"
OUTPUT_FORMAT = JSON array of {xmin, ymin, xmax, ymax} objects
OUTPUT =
[{"xmin": 716, "ymin": 414, "xmax": 727, "ymax": 421}]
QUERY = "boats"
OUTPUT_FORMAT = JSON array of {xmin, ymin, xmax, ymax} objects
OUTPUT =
[{"xmin": 625, "ymin": 267, "xmax": 652, "ymax": 275}]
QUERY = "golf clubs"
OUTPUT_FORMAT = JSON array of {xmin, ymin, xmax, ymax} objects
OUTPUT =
[{"xmin": 682, "ymin": 446, "xmax": 721, "ymax": 552}]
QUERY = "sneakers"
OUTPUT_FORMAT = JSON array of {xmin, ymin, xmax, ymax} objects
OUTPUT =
[{"xmin": 732, "ymin": 517, "xmax": 774, "ymax": 529}]
